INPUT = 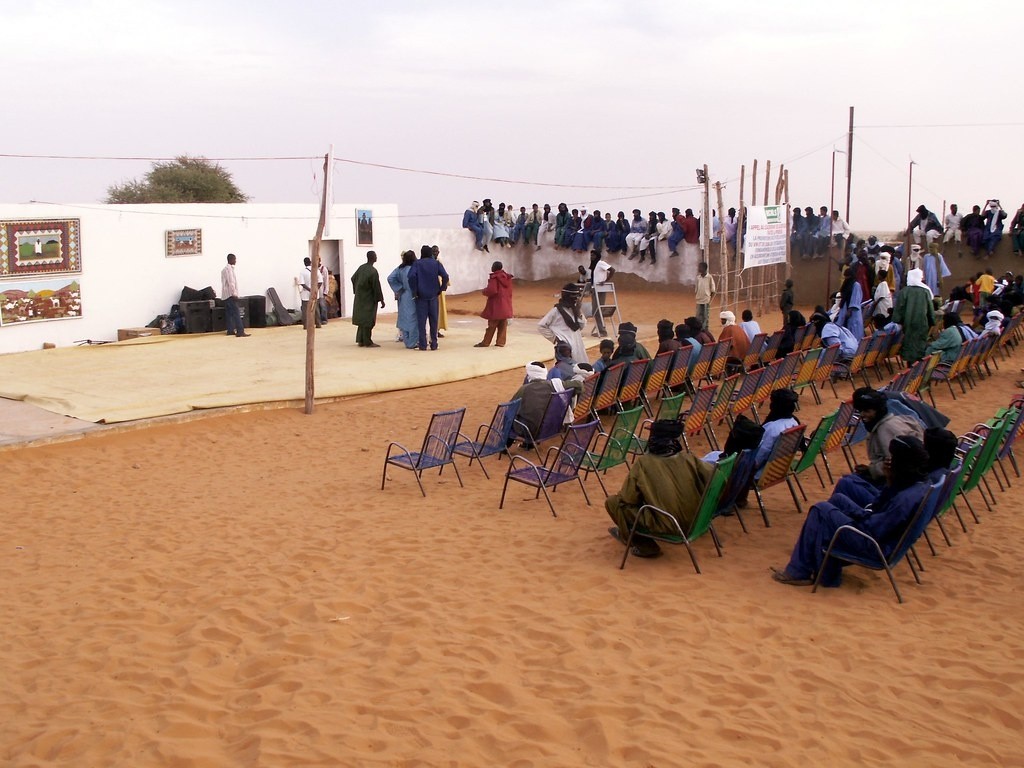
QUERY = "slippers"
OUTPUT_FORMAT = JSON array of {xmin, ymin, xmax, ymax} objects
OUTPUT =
[
  {"xmin": 633, "ymin": 546, "xmax": 659, "ymax": 558},
  {"xmin": 772, "ymin": 569, "xmax": 813, "ymax": 585},
  {"xmin": 608, "ymin": 525, "xmax": 627, "ymax": 544}
]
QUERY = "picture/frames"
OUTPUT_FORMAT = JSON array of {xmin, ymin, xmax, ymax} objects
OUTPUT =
[{"xmin": 355, "ymin": 207, "xmax": 373, "ymax": 247}]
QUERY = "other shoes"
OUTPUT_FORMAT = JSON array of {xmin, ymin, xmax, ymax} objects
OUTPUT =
[
  {"xmin": 475, "ymin": 343, "xmax": 485, "ymax": 347},
  {"xmin": 367, "ymin": 343, "xmax": 380, "ymax": 347},
  {"xmin": 438, "ymin": 332, "xmax": 444, "ymax": 338},
  {"xmin": 941, "ymin": 250, "xmax": 1024, "ymax": 260},
  {"xmin": 592, "ymin": 333, "xmax": 607, "ymax": 337},
  {"xmin": 237, "ymin": 333, "xmax": 250, "ymax": 337},
  {"xmin": 518, "ymin": 443, "xmax": 535, "ymax": 450},
  {"xmin": 500, "ymin": 241, "xmax": 679, "ymax": 265},
  {"xmin": 228, "ymin": 332, "xmax": 236, "ymax": 334},
  {"xmin": 322, "ymin": 321, "xmax": 327, "ymax": 324},
  {"xmin": 800, "ymin": 252, "xmax": 826, "ymax": 261}
]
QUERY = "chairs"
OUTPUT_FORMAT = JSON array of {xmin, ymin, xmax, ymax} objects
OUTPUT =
[
  {"xmin": 381, "ymin": 300, "xmax": 1024, "ymax": 605},
  {"xmin": 579, "ymin": 283, "xmax": 623, "ymax": 337}
]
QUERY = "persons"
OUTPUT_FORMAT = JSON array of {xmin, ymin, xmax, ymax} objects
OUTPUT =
[
  {"xmin": 776, "ymin": 235, "xmax": 1024, "ymax": 376},
  {"xmin": 537, "ymin": 282, "xmax": 588, "ymax": 364},
  {"xmin": 713, "ymin": 208, "xmax": 748, "ymax": 259},
  {"xmin": 474, "ymin": 262, "xmax": 515, "ymax": 347},
  {"xmin": 462, "ymin": 199, "xmax": 700, "ymax": 265},
  {"xmin": 299, "ymin": 256, "xmax": 338, "ymax": 330},
  {"xmin": 694, "ymin": 262, "xmax": 717, "ymax": 330},
  {"xmin": 790, "ymin": 206, "xmax": 851, "ymax": 261},
  {"xmin": 577, "ymin": 266, "xmax": 592, "ymax": 284},
  {"xmin": 588, "ymin": 249, "xmax": 615, "ymax": 338},
  {"xmin": 220, "ymin": 253, "xmax": 252, "ymax": 337},
  {"xmin": 773, "ymin": 387, "xmax": 958, "ymax": 589},
  {"xmin": 604, "ymin": 388, "xmax": 801, "ymax": 560},
  {"xmin": 387, "ymin": 245, "xmax": 449, "ymax": 351},
  {"xmin": 351, "ymin": 251, "xmax": 385, "ymax": 348},
  {"xmin": 510, "ymin": 309, "xmax": 762, "ymax": 454},
  {"xmin": 911, "ymin": 200, "xmax": 1024, "ymax": 261}
]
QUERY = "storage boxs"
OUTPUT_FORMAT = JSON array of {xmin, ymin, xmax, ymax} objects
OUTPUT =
[{"xmin": 118, "ymin": 328, "xmax": 162, "ymax": 341}]
